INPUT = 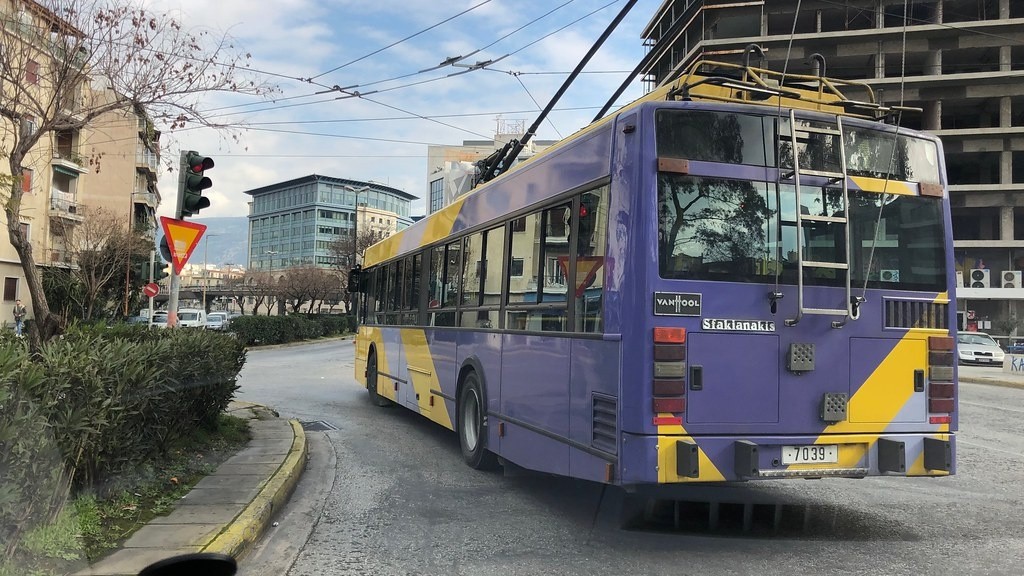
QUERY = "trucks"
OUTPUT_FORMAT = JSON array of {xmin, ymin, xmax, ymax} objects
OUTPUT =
[
  {"xmin": 177, "ymin": 309, "xmax": 206, "ymax": 331},
  {"xmin": 209, "ymin": 312, "xmax": 230, "ymax": 331}
]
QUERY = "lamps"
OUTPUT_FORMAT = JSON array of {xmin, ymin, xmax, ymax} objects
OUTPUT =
[{"xmin": 880, "ymin": 270, "xmax": 899, "ymax": 282}]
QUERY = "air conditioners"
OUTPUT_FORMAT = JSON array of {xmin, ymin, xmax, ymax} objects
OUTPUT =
[
  {"xmin": 955, "ymin": 271, "xmax": 964, "ymax": 288},
  {"xmin": 970, "ymin": 269, "xmax": 990, "ymax": 288},
  {"xmin": 1000, "ymin": 271, "xmax": 1022, "ymax": 288}
]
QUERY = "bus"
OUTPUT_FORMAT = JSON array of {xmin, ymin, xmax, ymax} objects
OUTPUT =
[{"xmin": 345, "ymin": 44, "xmax": 966, "ymax": 534}]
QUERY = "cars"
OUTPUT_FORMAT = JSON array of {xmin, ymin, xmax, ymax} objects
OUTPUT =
[
  {"xmin": 205, "ymin": 315, "xmax": 223, "ymax": 332},
  {"xmin": 152, "ymin": 313, "xmax": 179, "ymax": 329},
  {"xmin": 956, "ymin": 331, "xmax": 1005, "ymax": 367},
  {"xmin": 124, "ymin": 315, "xmax": 148, "ymax": 329}
]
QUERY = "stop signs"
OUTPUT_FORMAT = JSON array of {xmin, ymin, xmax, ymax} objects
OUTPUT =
[{"xmin": 144, "ymin": 283, "xmax": 160, "ymax": 297}]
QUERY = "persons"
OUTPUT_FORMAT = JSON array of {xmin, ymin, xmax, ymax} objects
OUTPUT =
[{"xmin": 12, "ymin": 300, "xmax": 27, "ymax": 337}]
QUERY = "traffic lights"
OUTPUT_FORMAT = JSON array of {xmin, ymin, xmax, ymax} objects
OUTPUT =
[{"xmin": 181, "ymin": 150, "xmax": 215, "ymax": 215}]
